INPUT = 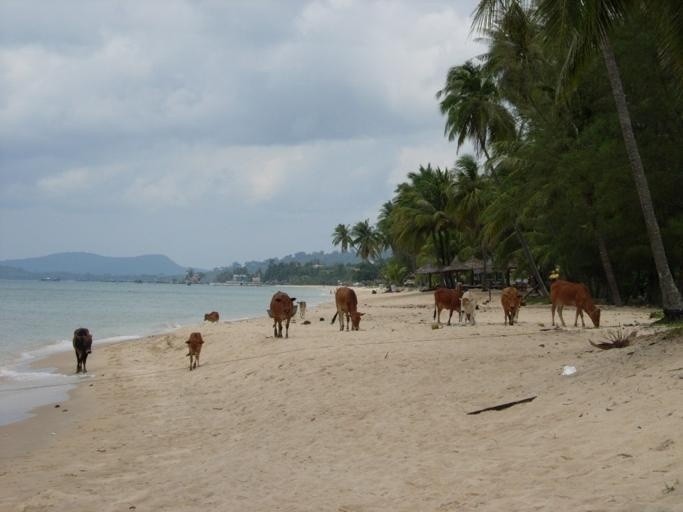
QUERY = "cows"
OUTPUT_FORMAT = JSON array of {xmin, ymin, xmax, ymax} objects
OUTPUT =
[
  {"xmin": 432, "ymin": 287, "xmax": 478, "ymax": 326},
  {"xmin": 185, "ymin": 332, "xmax": 205, "ymax": 371},
  {"xmin": 499, "ymin": 286, "xmax": 523, "ymax": 327},
  {"xmin": 329, "ymin": 286, "xmax": 366, "ymax": 331},
  {"xmin": 203, "ymin": 311, "xmax": 220, "ymax": 323},
  {"xmin": 297, "ymin": 300, "xmax": 309, "ymax": 319},
  {"xmin": 549, "ymin": 279, "xmax": 603, "ymax": 328},
  {"xmin": 72, "ymin": 326, "xmax": 93, "ymax": 374},
  {"xmin": 269, "ymin": 290, "xmax": 297, "ymax": 338}
]
